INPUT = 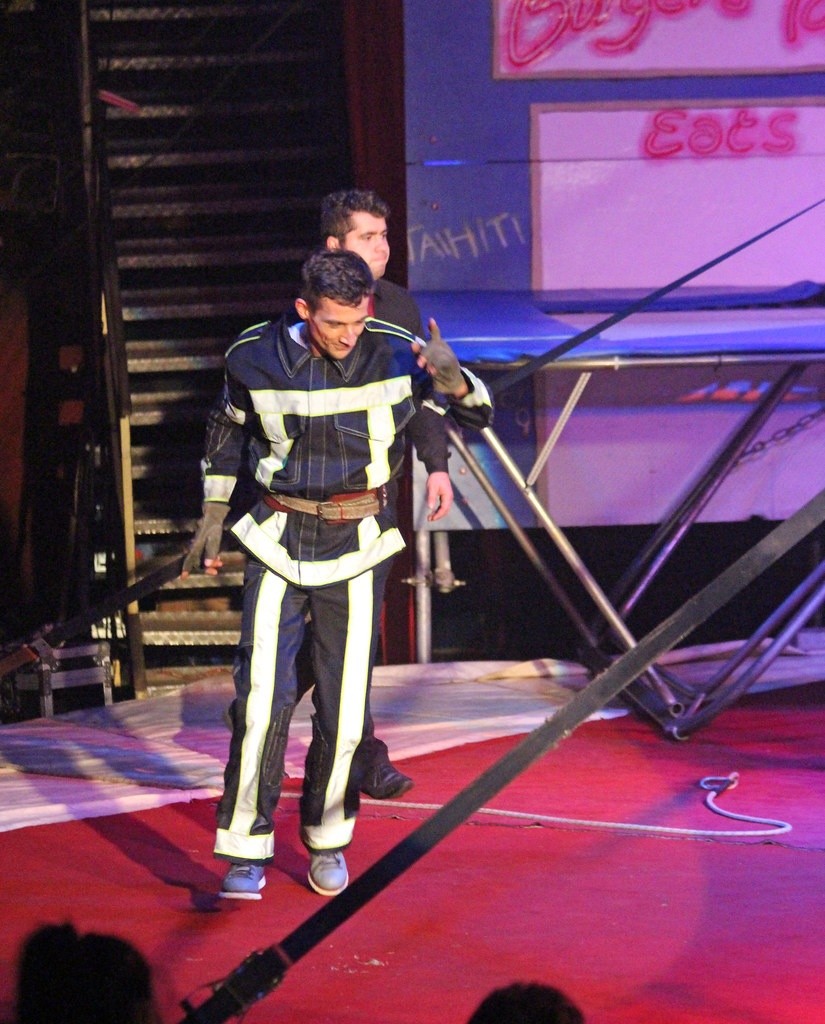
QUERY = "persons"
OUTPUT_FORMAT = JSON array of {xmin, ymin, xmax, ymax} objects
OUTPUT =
[
  {"xmin": 13, "ymin": 921, "xmax": 161, "ymax": 1024},
  {"xmin": 224, "ymin": 189, "xmax": 495, "ymax": 799},
  {"xmin": 179, "ymin": 249, "xmax": 454, "ymax": 899}
]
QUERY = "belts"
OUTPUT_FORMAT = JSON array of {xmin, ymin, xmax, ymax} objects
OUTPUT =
[{"xmin": 271, "ymin": 493, "xmax": 380, "ymax": 520}]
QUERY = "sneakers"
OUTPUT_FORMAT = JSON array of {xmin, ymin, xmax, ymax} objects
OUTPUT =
[
  {"xmin": 218, "ymin": 865, "xmax": 267, "ymax": 900},
  {"xmin": 361, "ymin": 763, "xmax": 414, "ymax": 799},
  {"xmin": 307, "ymin": 852, "xmax": 349, "ymax": 896}
]
